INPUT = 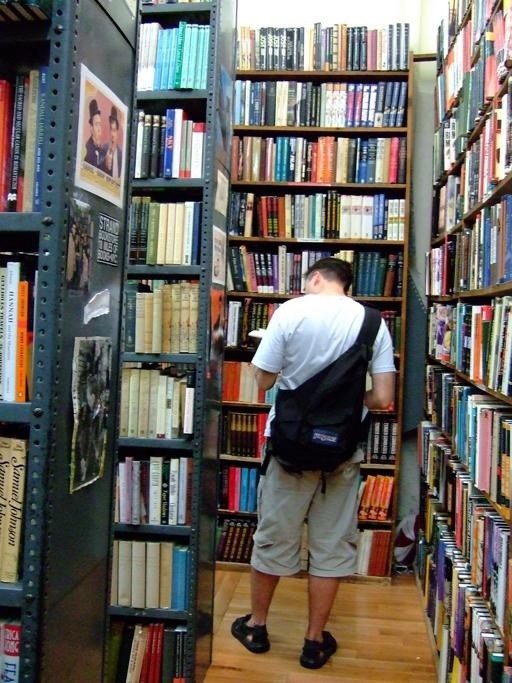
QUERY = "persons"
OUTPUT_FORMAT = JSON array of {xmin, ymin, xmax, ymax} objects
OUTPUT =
[
  {"xmin": 73, "ymin": 358, "xmax": 109, "ymax": 477},
  {"xmin": 83, "ymin": 101, "xmax": 124, "ymax": 188},
  {"xmin": 230, "ymin": 258, "xmax": 395, "ymax": 671},
  {"xmin": 67, "ymin": 204, "xmax": 89, "ymax": 289}
]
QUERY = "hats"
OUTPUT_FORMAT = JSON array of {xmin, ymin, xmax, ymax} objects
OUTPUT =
[
  {"xmin": 89, "ymin": 99, "xmax": 100, "ymax": 123},
  {"xmin": 109, "ymin": 105, "xmax": 119, "ymax": 129}
]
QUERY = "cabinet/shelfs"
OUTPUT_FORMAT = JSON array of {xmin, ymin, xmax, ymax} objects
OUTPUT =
[
  {"xmin": 214, "ymin": 51, "xmax": 416, "ymax": 589},
  {"xmin": 100, "ymin": 0, "xmax": 240, "ymax": 678},
  {"xmin": 1, "ymin": 2, "xmax": 145, "ymax": 677},
  {"xmin": 416, "ymin": 3, "xmax": 512, "ymax": 678}
]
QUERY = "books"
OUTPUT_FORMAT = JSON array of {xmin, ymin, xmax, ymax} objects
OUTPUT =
[
  {"xmin": 222, "ymin": 361, "xmax": 277, "ymax": 560},
  {"xmin": 119, "ymin": 454, "xmax": 194, "ymax": 525},
  {"xmin": 0, "ymin": 18, "xmax": 54, "ymax": 682},
  {"xmin": 119, "ymin": 362, "xmax": 196, "ymax": 441},
  {"xmin": 222, "ymin": 247, "xmax": 277, "ymax": 560},
  {"xmin": 118, "ymin": 623, "xmax": 187, "ymax": 683},
  {"xmin": 418, "ymin": 1, "xmax": 511, "ymax": 683},
  {"xmin": 358, "ymin": 382, "xmax": 396, "ymax": 582},
  {"xmin": 128, "ymin": 197, "xmax": 202, "ymax": 265},
  {"xmin": 218, "ymin": 246, "xmax": 399, "ymax": 370},
  {"xmin": 110, "ymin": 540, "xmax": 190, "ymax": 610},
  {"xmin": 125, "ymin": 279, "xmax": 199, "ymax": 353},
  {"xmin": 234, "ymin": 21, "xmax": 410, "ymax": 239},
  {"xmin": 138, "ymin": 21, "xmax": 210, "ymax": 91},
  {"xmin": 135, "ymin": 109, "xmax": 205, "ymax": 179}
]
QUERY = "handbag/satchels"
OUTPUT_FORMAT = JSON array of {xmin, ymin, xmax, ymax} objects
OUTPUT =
[{"xmin": 271, "ymin": 344, "xmax": 372, "ymax": 472}]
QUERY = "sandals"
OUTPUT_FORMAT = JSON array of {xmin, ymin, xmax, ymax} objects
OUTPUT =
[
  {"xmin": 231, "ymin": 614, "xmax": 269, "ymax": 653},
  {"xmin": 300, "ymin": 631, "xmax": 336, "ymax": 669}
]
